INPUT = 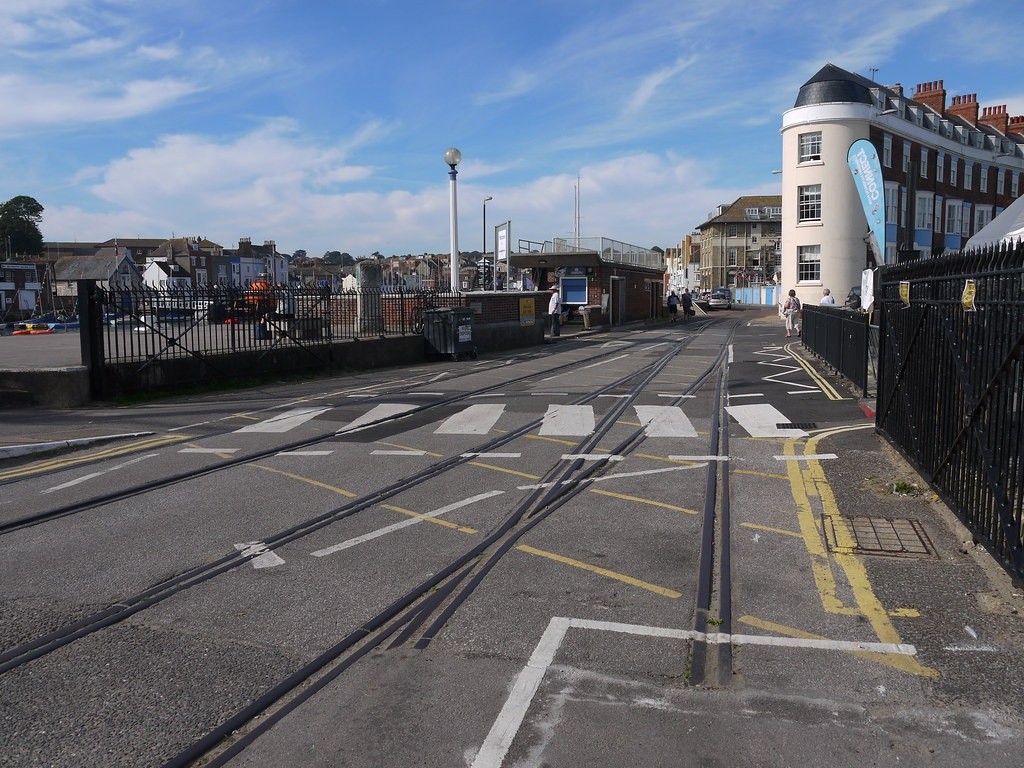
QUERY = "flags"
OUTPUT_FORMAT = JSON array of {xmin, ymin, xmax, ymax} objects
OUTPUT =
[{"xmin": 734, "ymin": 267, "xmax": 752, "ymax": 284}]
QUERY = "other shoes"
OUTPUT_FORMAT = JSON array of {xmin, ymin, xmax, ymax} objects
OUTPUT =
[
  {"xmin": 797, "ymin": 330, "xmax": 801, "ymax": 336},
  {"xmin": 674, "ymin": 318, "xmax": 676, "ymax": 322},
  {"xmin": 785, "ymin": 335, "xmax": 792, "ymax": 338},
  {"xmin": 671, "ymin": 320, "xmax": 673, "ymax": 323}
]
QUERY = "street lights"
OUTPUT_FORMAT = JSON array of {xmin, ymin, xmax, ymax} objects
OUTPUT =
[
  {"xmin": 445, "ymin": 148, "xmax": 462, "ymax": 294},
  {"xmin": 483, "ymin": 196, "xmax": 493, "ymax": 291}
]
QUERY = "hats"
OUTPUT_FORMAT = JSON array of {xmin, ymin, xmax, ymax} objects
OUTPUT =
[{"xmin": 549, "ymin": 285, "xmax": 557, "ymax": 290}]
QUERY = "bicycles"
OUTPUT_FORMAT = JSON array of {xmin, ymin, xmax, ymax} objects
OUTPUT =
[{"xmin": 408, "ymin": 294, "xmax": 437, "ymax": 334}]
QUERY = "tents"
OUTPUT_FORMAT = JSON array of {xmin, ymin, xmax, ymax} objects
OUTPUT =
[{"xmin": 963, "ymin": 195, "xmax": 1024, "ymax": 255}]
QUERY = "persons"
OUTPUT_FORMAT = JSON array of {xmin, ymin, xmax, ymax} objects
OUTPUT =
[
  {"xmin": 258, "ymin": 282, "xmax": 296, "ymax": 340},
  {"xmin": 489, "ymin": 271, "xmax": 504, "ymax": 289},
  {"xmin": 666, "ymin": 290, "xmax": 681, "ymax": 323},
  {"xmin": 547, "ymin": 284, "xmax": 563, "ymax": 338},
  {"xmin": 681, "ymin": 288, "xmax": 696, "ymax": 323},
  {"xmin": 782, "ymin": 289, "xmax": 801, "ymax": 338},
  {"xmin": 316, "ymin": 280, "xmax": 332, "ymax": 296},
  {"xmin": 817, "ymin": 288, "xmax": 833, "ymax": 306}
]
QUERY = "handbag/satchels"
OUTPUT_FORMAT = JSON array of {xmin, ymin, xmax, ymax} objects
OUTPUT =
[{"xmin": 690, "ymin": 305, "xmax": 695, "ymax": 315}]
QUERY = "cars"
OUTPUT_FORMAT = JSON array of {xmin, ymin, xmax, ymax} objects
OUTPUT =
[{"xmin": 697, "ymin": 286, "xmax": 732, "ymax": 310}]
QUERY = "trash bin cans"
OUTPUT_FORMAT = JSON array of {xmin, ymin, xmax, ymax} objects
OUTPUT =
[{"xmin": 421, "ymin": 306, "xmax": 477, "ymax": 362}]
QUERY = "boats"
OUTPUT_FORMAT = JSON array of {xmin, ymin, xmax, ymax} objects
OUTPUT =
[{"xmin": 0, "ymin": 234, "xmax": 296, "ymax": 333}]
